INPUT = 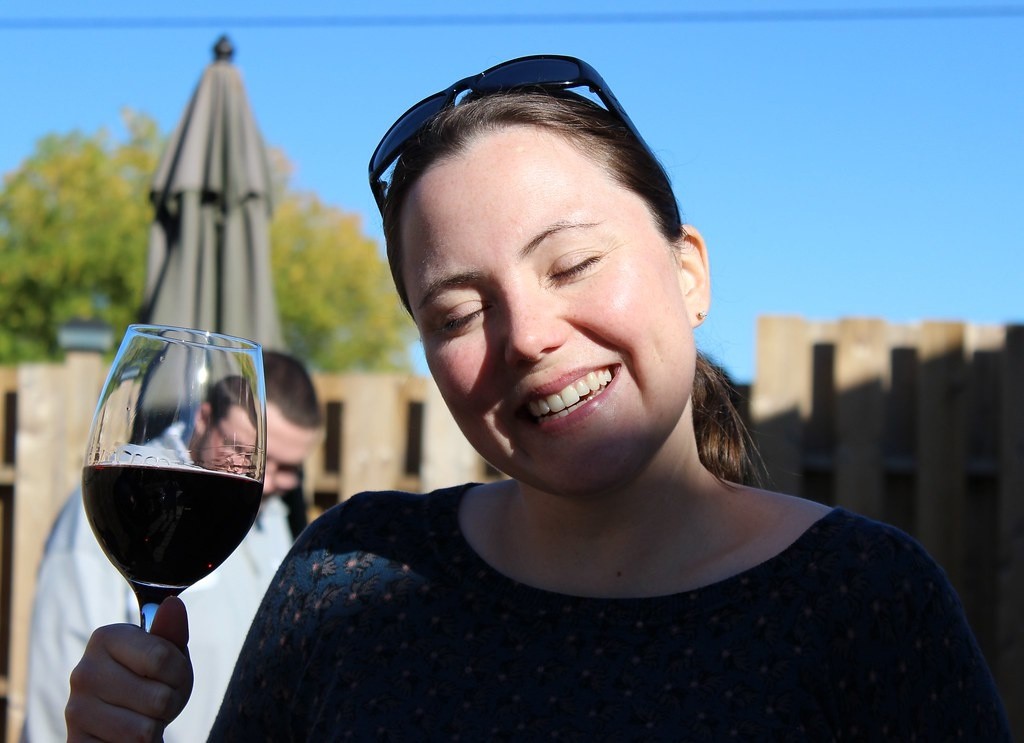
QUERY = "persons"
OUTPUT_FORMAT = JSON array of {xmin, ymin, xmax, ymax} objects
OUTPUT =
[
  {"xmin": 17, "ymin": 347, "xmax": 327, "ymax": 743},
  {"xmin": 63, "ymin": 54, "xmax": 1013, "ymax": 743}
]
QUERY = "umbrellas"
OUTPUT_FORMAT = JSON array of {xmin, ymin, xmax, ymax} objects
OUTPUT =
[{"xmin": 142, "ymin": 33, "xmax": 296, "ymax": 410}]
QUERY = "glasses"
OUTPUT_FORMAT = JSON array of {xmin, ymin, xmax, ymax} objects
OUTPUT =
[
  {"xmin": 368, "ymin": 55, "xmax": 644, "ymax": 220},
  {"xmin": 213, "ymin": 420, "xmax": 305, "ymax": 489}
]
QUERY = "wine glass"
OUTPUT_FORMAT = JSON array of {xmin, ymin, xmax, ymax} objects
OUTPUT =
[{"xmin": 81, "ymin": 324, "xmax": 267, "ymax": 631}]
